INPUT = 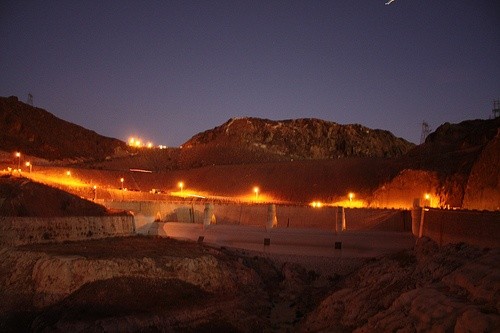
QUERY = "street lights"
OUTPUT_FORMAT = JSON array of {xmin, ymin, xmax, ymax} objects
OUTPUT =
[
  {"xmin": 26, "ymin": 162, "xmax": 32, "ymax": 174},
  {"xmin": 179, "ymin": 182, "xmax": 183, "ymax": 192},
  {"xmin": 16, "ymin": 152, "xmax": 20, "ymax": 169},
  {"xmin": 121, "ymin": 178, "xmax": 123, "ymax": 188}
]
